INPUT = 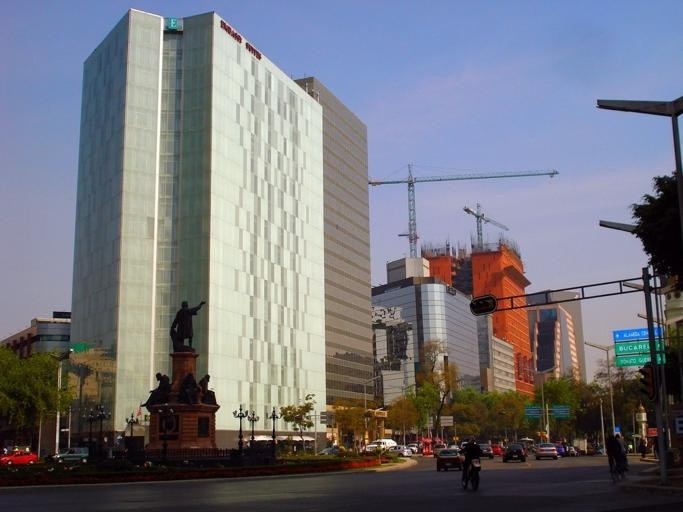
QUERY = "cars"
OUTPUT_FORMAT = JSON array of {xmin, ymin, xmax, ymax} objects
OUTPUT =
[
  {"xmin": 0, "ymin": 449, "xmax": 37, "ymax": 465},
  {"xmin": 314, "ymin": 447, "xmax": 345, "ymax": 457},
  {"xmin": 434, "ymin": 447, "xmax": 464, "ymax": 472},
  {"xmin": 361, "ymin": 438, "xmax": 604, "ymax": 462}
]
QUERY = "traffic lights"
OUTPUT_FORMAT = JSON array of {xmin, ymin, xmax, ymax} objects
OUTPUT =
[
  {"xmin": 469, "ymin": 293, "xmax": 499, "ymax": 317},
  {"xmin": 637, "ymin": 363, "xmax": 654, "ymax": 400}
]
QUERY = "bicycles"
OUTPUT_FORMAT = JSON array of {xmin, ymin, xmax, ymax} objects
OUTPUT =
[{"xmin": 607, "ymin": 456, "xmax": 622, "ymax": 485}]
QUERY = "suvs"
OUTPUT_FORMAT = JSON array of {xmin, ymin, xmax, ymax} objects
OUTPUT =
[{"xmin": 44, "ymin": 446, "xmax": 89, "ymax": 465}]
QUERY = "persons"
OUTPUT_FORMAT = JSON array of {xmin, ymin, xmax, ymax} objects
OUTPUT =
[
  {"xmin": 141, "ymin": 301, "xmax": 216, "ymax": 405},
  {"xmin": 608, "ymin": 433, "xmax": 639, "ymax": 483},
  {"xmin": 462, "ymin": 438, "xmax": 480, "ymax": 486}
]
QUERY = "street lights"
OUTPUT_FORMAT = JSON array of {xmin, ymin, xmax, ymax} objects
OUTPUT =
[
  {"xmin": 618, "ymin": 276, "xmax": 681, "ymax": 337},
  {"xmin": 362, "ymin": 375, "xmax": 381, "ymax": 440},
  {"xmin": 125, "ymin": 413, "xmax": 139, "ymax": 436},
  {"xmin": 399, "ymin": 382, "xmax": 417, "ymax": 444},
  {"xmin": 591, "ymin": 93, "xmax": 681, "ymax": 240},
  {"xmin": 582, "ymin": 340, "xmax": 615, "ymax": 430},
  {"xmin": 517, "ymin": 364, "xmax": 560, "ymax": 443},
  {"xmin": 80, "ymin": 408, "xmax": 98, "ymax": 445},
  {"xmin": 594, "ymin": 214, "xmax": 638, "ymax": 239},
  {"xmin": 246, "ymin": 410, "xmax": 259, "ymax": 440},
  {"xmin": 91, "ymin": 403, "xmax": 111, "ymax": 448},
  {"xmin": 48, "ymin": 347, "xmax": 74, "ymax": 452},
  {"xmin": 634, "ymin": 312, "xmax": 683, "ymax": 348},
  {"xmin": 157, "ymin": 403, "xmax": 173, "ymax": 460},
  {"xmin": 365, "ymin": 407, "xmax": 383, "ymax": 427},
  {"xmin": 231, "ymin": 404, "xmax": 249, "ymax": 452},
  {"xmin": 264, "ymin": 406, "xmax": 283, "ymax": 440}
]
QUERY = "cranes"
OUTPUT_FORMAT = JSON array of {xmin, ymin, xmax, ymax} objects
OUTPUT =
[
  {"xmin": 369, "ymin": 162, "xmax": 559, "ymax": 251},
  {"xmin": 457, "ymin": 196, "xmax": 512, "ymax": 246}
]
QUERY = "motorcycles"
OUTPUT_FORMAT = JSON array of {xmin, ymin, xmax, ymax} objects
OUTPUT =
[{"xmin": 458, "ymin": 448, "xmax": 480, "ymax": 491}]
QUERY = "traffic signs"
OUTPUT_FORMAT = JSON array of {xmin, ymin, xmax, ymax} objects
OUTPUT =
[
  {"xmin": 612, "ymin": 326, "xmax": 664, "ymax": 343},
  {"xmin": 611, "ymin": 353, "xmax": 667, "ymax": 369},
  {"xmin": 612, "ymin": 341, "xmax": 665, "ymax": 356}
]
